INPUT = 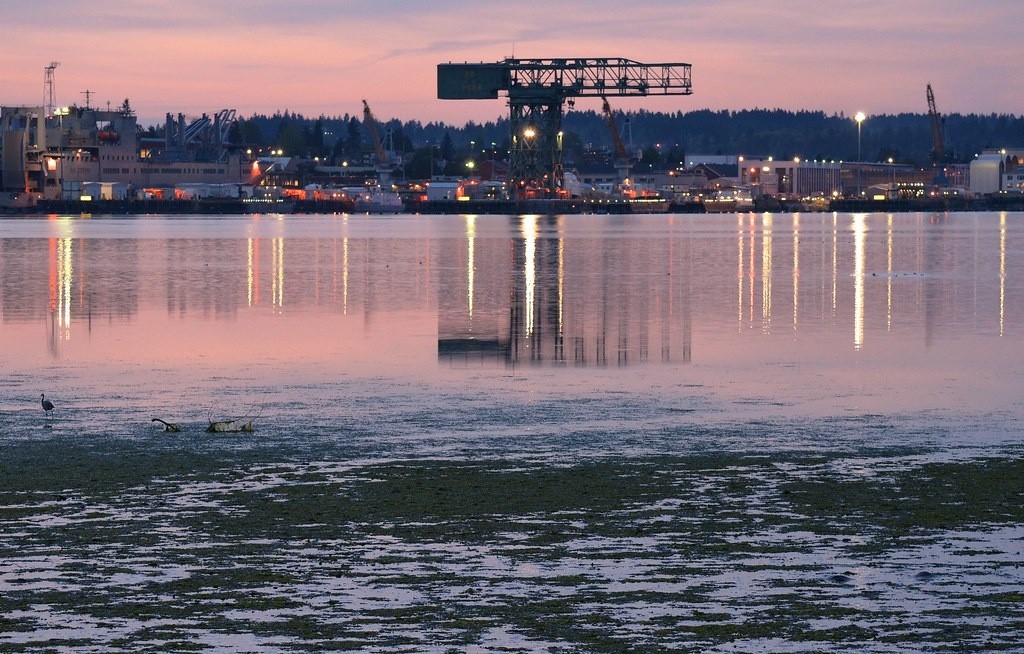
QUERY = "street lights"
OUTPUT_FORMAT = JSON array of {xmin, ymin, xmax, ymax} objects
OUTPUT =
[{"xmin": 854, "ymin": 112, "xmax": 866, "ymax": 198}]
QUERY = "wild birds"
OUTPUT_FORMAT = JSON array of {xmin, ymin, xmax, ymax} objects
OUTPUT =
[{"xmin": 39, "ymin": 394, "xmax": 55, "ymax": 416}]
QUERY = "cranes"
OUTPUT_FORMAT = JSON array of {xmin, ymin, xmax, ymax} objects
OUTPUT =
[
  {"xmin": 926, "ymin": 83, "xmax": 951, "ymax": 186},
  {"xmin": 597, "ymin": 95, "xmax": 631, "ymax": 165},
  {"xmin": 361, "ymin": 98, "xmax": 391, "ymax": 172}
]
